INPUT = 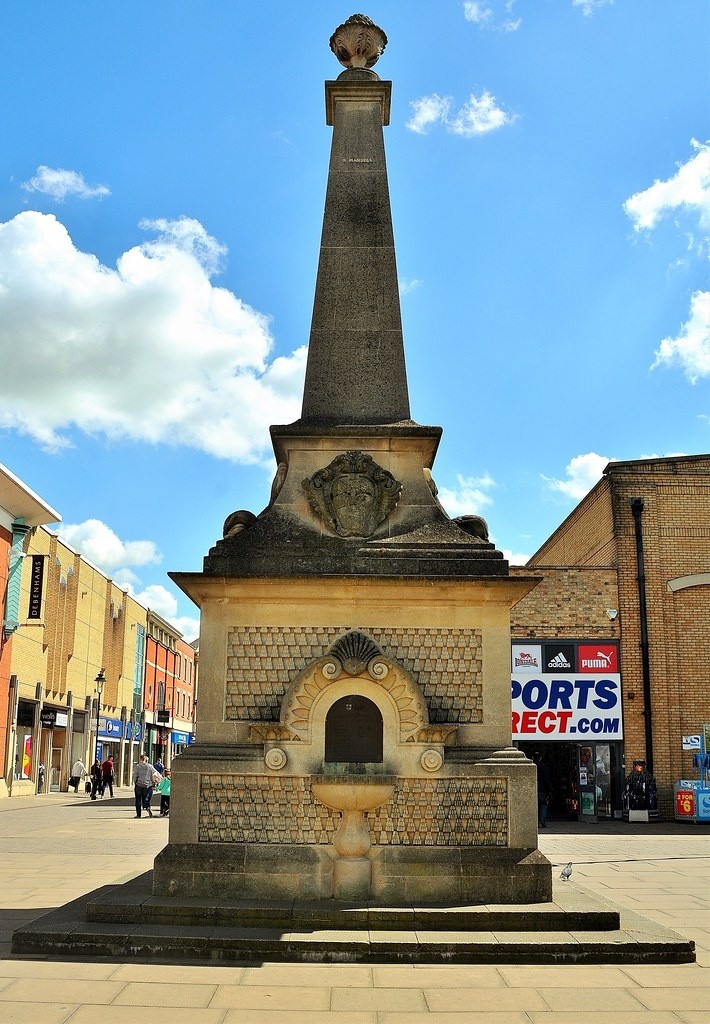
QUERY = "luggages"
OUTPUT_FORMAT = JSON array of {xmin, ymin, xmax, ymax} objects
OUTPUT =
[{"xmin": 85, "ymin": 782, "xmax": 92, "ymax": 794}]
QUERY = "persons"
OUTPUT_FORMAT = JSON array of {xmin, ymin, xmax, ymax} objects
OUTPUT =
[
  {"xmin": 152, "ymin": 758, "xmax": 164, "ymax": 792},
  {"xmin": 100, "ymin": 755, "xmax": 115, "ymax": 799},
  {"xmin": 152, "ymin": 769, "xmax": 171, "ymax": 817},
  {"xmin": 132, "ymin": 755, "xmax": 163, "ymax": 819},
  {"xmin": 531, "ymin": 751, "xmax": 555, "ymax": 828},
  {"xmin": 90, "ymin": 759, "xmax": 103, "ymax": 800},
  {"xmin": 72, "ymin": 757, "xmax": 88, "ymax": 793}
]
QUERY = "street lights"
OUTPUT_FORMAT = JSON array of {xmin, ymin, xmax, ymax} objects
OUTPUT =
[{"xmin": 94, "ymin": 671, "xmax": 107, "ymax": 761}]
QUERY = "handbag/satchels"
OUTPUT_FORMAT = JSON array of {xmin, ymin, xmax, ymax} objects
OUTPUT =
[{"xmin": 68, "ymin": 777, "xmax": 74, "ymax": 787}]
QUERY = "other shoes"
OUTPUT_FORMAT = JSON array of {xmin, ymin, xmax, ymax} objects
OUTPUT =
[
  {"xmin": 134, "ymin": 816, "xmax": 141, "ymax": 818},
  {"xmin": 101, "ymin": 795, "xmax": 103, "ymax": 798},
  {"xmin": 110, "ymin": 795, "xmax": 115, "ymax": 798},
  {"xmin": 74, "ymin": 790, "xmax": 78, "ymax": 793},
  {"xmin": 540, "ymin": 821, "xmax": 546, "ymax": 828},
  {"xmin": 149, "ymin": 809, "xmax": 152, "ymax": 817}
]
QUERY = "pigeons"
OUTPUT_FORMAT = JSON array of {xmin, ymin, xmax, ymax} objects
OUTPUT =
[{"xmin": 559, "ymin": 862, "xmax": 573, "ymax": 879}]
